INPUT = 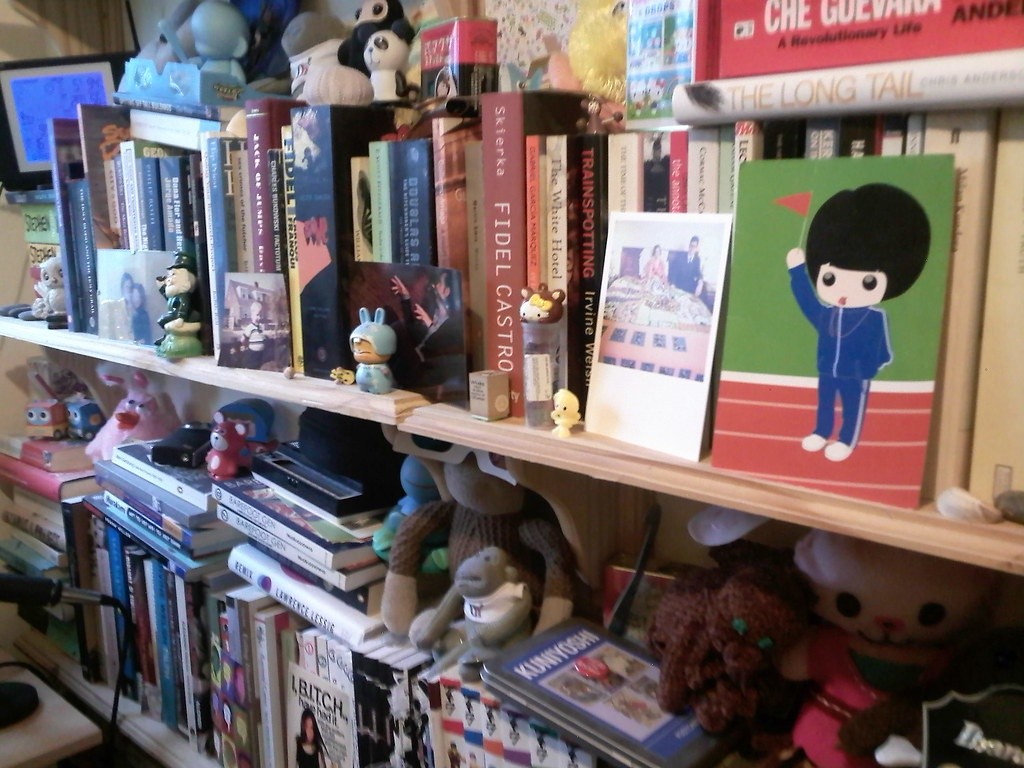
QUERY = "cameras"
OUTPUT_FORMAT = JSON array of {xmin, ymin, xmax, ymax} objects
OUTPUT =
[{"xmin": 151, "ymin": 421, "xmax": 215, "ymax": 468}]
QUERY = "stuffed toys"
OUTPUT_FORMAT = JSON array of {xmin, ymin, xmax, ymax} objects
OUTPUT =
[
  {"xmin": 642, "ymin": 560, "xmax": 809, "ymax": 735},
  {"xmin": 369, "ymin": 450, "xmax": 454, "ymax": 591},
  {"xmin": 547, "ymin": 0, "xmax": 630, "ymax": 133},
  {"xmin": 382, "ymin": 450, "xmax": 590, "ymax": 641},
  {"xmin": 415, "ymin": 544, "xmax": 533, "ymax": 680},
  {"xmin": 775, "ymin": 527, "xmax": 1005, "ymax": 768}
]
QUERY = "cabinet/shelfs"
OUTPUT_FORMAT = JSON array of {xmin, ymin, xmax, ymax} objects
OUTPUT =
[{"xmin": 0, "ymin": 310, "xmax": 1024, "ymax": 768}]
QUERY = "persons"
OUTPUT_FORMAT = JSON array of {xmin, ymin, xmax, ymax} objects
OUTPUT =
[
  {"xmin": 647, "ymin": 137, "xmax": 669, "ymax": 198},
  {"xmin": 130, "ymin": 282, "xmax": 152, "ymax": 347},
  {"xmin": 294, "ymin": 701, "xmax": 333, "ymax": 768},
  {"xmin": 391, "ymin": 270, "xmax": 464, "ymax": 391},
  {"xmin": 669, "ymin": 236, "xmax": 713, "ymax": 315},
  {"xmin": 118, "ymin": 270, "xmax": 137, "ymax": 319},
  {"xmin": 186, "ymin": 601, "xmax": 208, "ymax": 677},
  {"xmin": 239, "ymin": 302, "xmax": 268, "ymax": 371},
  {"xmin": 641, "ymin": 242, "xmax": 664, "ymax": 288},
  {"xmin": 447, "ymin": 743, "xmax": 460, "ymax": 768}
]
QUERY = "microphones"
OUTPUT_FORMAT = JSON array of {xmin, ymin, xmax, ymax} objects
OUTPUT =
[{"xmin": 0, "ymin": 573, "xmax": 120, "ymax": 608}]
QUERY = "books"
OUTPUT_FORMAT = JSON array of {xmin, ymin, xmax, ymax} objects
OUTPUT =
[
  {"xmin": 21, "ymin": 0, "xmax": 1024, "ymax": 519},
  {"xmin": 0, "ymin": 428, "xmax": 768, "ymax": 768}
]
